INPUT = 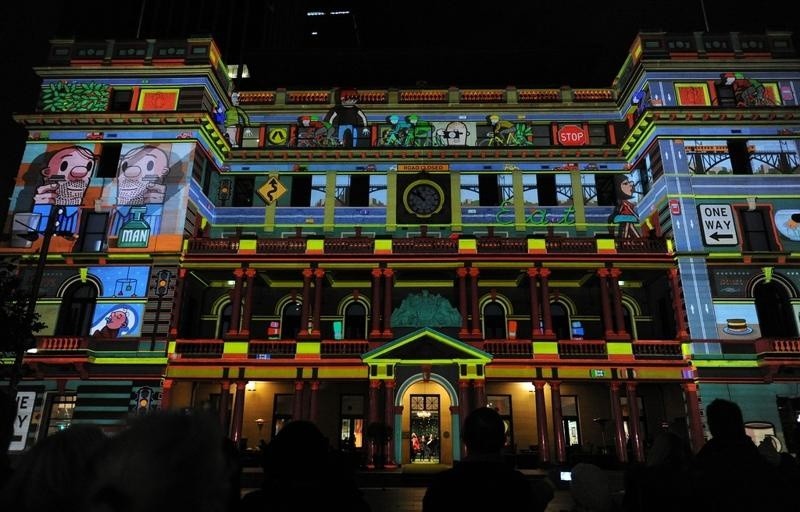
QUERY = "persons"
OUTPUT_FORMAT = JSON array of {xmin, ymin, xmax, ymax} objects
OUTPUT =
[
  {"xmin": 1, "ymin": 396, "xmax": 372, "ymax": 511},
  {"xmin": 571, "ymin": 400, "xmax": 797, "ymax": 510},
  {"xmin": 411, "ymin": 433, "xmax": 439, "ymax": 461},
  {"xmin": 423, "ymin": 407, "xmax": 554, "ymax": 510}
]
